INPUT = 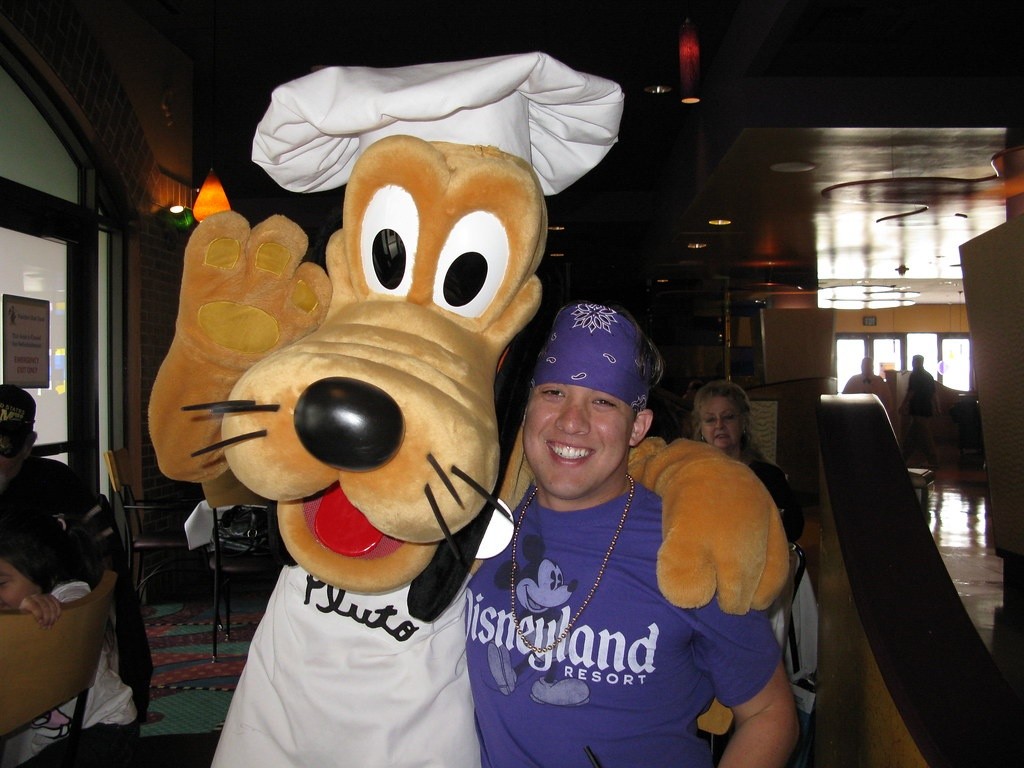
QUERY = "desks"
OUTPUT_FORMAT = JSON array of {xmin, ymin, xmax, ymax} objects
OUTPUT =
[{"xmin": 183, "ymin": 499, "xmax": 269, "ymax": 551}]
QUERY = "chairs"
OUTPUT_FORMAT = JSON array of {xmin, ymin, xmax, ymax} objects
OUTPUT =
[
  {"xmin": 212, "ymin": 501, "xmax": 284, "ymax": 660},
  {"xmin": 104, "ymin": 445, "xmax": 212, "ymax": 606},
  {"xmin": 0, "ymin": 570, "xmax": 118, "ymax": 766}
]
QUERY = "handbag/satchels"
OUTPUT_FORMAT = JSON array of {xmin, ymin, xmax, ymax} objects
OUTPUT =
[{"xmin": 209, "ymin": 503, "xmax": 284, "ymax": 559}]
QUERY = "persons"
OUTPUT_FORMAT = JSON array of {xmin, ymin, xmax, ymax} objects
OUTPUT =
[
  {"xmin": 692, "ymin": 381, "xmax": 805, "ymax": 653},
  {"xmin": 898, "ymin": 356, "xmax": 940, "ymax": 469},
  {"xmin": 0, "ymin": 385, "xmax": 129, "ymax": 768},
  {"xmin": 842, "ymin": 358, "xmax": 893, "ymax": 415},
  {"xmin": 462, "ymin": 300, "xmax": 799, "ymax": 768}
]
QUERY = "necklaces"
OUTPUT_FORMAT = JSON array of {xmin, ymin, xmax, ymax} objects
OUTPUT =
[{"xmin": 511, "ymin": 472, "xmax": 634, "ymax": 653}]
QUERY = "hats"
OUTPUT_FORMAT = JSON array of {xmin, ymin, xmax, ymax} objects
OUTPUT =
[{"xmin": 0, "ymin": 384, "xmax": 36, "ymax": 457}]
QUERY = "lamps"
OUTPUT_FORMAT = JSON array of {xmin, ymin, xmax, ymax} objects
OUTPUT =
[
  {"xmin": 678, "ymin": 18, "xmax": 701, "ymax": 104},
  {"xmin": 191, "ymin": 166, "xmax": 231, "ymax": 222}
]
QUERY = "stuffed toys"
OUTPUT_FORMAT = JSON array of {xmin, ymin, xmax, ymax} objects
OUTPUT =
[{"xmin": 148, "ymin": 52, "xmax": 789, "ymax": 768}]
